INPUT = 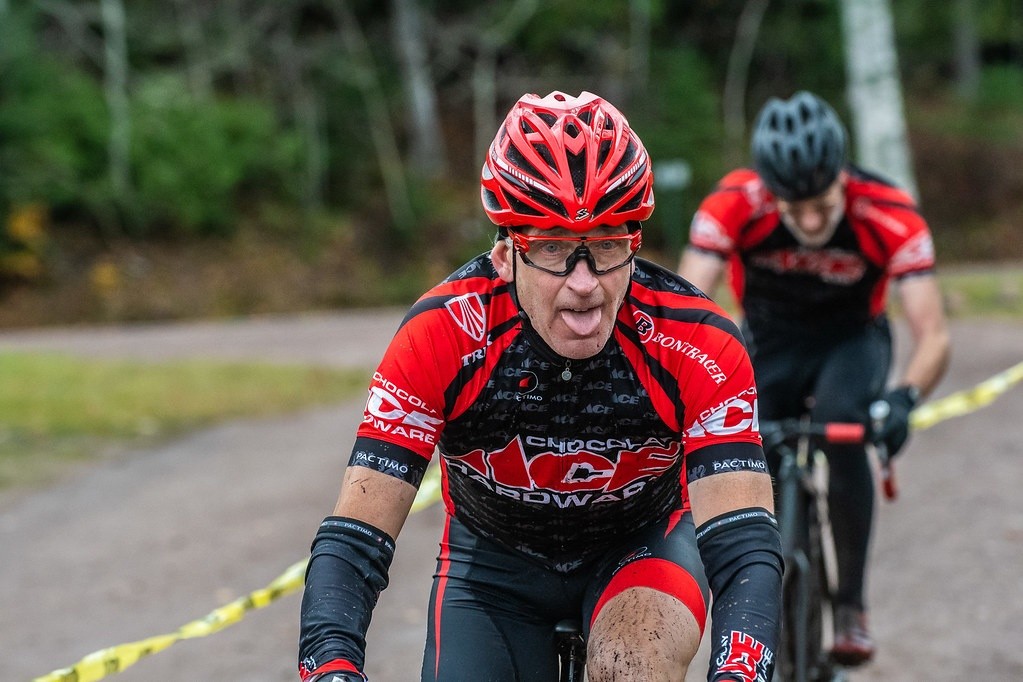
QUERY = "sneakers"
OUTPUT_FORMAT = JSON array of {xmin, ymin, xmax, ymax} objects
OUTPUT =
[{"xmin": 830, "ymin": 605, "xmax": 873, "ymax": 666}]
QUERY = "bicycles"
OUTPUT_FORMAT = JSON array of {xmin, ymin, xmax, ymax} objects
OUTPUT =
[{"xmin": 747, "ymin": 398, "xmax": 898, "ymax": 682}]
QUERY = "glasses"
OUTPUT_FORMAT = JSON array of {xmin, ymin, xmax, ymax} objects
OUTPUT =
[{"xmin": 506, "ymin": 228, "xmax": 641, "ymax": 278}]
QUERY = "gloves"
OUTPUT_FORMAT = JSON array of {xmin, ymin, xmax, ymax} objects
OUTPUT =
[{"xmin": 856, "ymin": 388, "xmax": 912, "ymax": 460}]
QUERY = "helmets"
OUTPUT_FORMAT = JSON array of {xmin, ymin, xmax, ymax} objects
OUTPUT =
[
  {"xmin": 754, "ymin": 91, "xmax": 845, "ymax": 199},
  {"xmin": 480, "ymin": 90, "xmax": 657, "ymax": 234}
]
